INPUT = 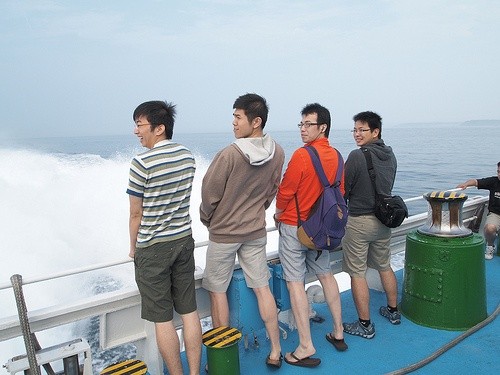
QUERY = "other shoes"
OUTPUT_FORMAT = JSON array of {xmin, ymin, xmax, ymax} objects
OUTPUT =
[{"xmin": 266, "ymin": 351, "xmax": 283, "ymax": 368}]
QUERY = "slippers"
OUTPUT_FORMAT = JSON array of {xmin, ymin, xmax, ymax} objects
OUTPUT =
[
  {"xmin": 326, "ymin": 331, "xmax": 348, "ymax": 351},
  {"xmin": 285, "ymin": 351, "xmax": 321, "ymax": 367}
]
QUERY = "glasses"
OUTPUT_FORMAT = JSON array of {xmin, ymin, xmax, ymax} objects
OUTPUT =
[
  {"xmin": 351, "ymin": 128, "xmax": 372, "ymax": 133},
  {"xmin": 134, "ymin": 123, "xmax": 151, "ymax": 128},
  {"xmin": 298, "ymin": 122, "xmax": 318, "ymax": 127}
]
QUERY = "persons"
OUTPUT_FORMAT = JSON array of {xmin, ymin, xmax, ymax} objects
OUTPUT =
[
  {"xmin": 456, "ymin": 162, "xmax": 500, "ymax": 260},
  {"xmin": 200, "ymin": 94, "xmax": 285, "ymax": 375},
  {"xmin": 125, "ymin": 101, "xmax": 203, "ymax": 375},
  {"xmin": 275, "ymin": 103, "xmax": 348, "ymax": 367},
  {"xmin": 342, "ymin": 111, "xmax": 401, "ymax": 339}
]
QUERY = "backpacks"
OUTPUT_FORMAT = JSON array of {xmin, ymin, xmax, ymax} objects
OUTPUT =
[{"xmin": 294, "ymin": 145, "xmax": 349, "ymax": 250}]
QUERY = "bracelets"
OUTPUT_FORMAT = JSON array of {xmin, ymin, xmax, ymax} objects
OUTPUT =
[{"xmin": 273, "ymin": 214, "xmax": 280, "ymax": 222}]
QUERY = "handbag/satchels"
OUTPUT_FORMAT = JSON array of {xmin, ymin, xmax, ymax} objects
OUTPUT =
[{"xmin": 374, "ymin": 193, "xmax": 408, "ymax": 228}]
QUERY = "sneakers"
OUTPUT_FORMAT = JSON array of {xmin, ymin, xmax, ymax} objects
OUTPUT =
[
  {"xmin": 343, "ymin": 320, "xmax": 376, "ymax": 339},
  {"xmin": 380, "ymin": 306, "xmax": 401, "ymax": 324},
  {"xmin": 485, "ymin": 245, "xmax": 496, "ymax": 260}
]
